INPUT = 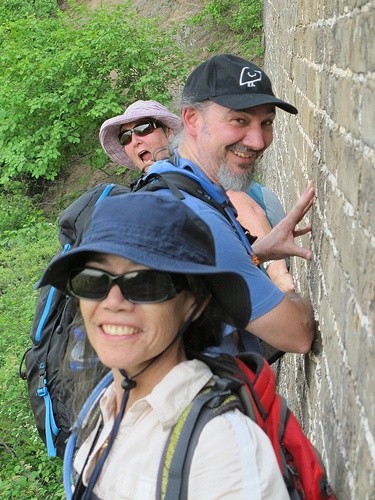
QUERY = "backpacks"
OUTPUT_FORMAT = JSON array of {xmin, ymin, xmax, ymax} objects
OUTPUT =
[
  {"xmin": 73, "ymin": 352, "xmax": 337, "ymax": 500},
  {"xmin": 245, "ymin": 179, "xmax": 291, "ymax": 272},
  {"xmin": 20, "ymin": 170, "xmax": 241, "ymax": 461}
]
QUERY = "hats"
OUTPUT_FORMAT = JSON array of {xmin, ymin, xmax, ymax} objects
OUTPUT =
[
  {"xmin": 98, "ymin": 100, "xmax": 182, "ymax": 171},
  {"xmin": 37, "ymin": 192, "xmax": 252, "ymax": 332},
  {"xmin": 182, "ymin": 55, "xmax": 298, "ymax": 114}
]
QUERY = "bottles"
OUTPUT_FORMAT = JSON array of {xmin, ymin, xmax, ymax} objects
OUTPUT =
[{"xmin": 66, "ymin": 329, "xmax": 99, "ymax": 372}]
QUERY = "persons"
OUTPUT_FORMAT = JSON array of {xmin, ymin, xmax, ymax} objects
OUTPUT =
[
  {"xmin": 99, "ymin": 54, "xmax": 318, "ymax": 362},
  {"xmin": 36, "ymin": 193, "xmax": 291, "ymax": 500}
]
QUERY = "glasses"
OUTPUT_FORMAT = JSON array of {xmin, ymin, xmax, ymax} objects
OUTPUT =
[
  {"xmin": 117, "ymin": 121, "xmax": 165, "ymax": 146},
  {"xmin": 67, "ymin": 264, "xmax": 197, "ymax": 304}
]
